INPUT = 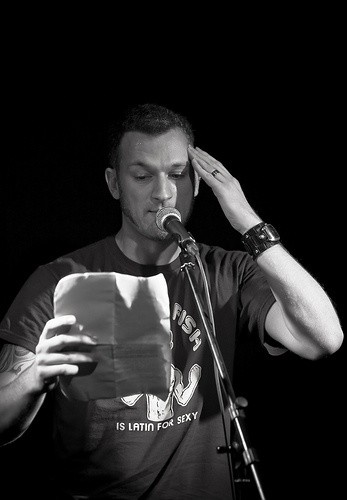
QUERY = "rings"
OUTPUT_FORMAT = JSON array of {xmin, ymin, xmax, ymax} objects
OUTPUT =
[{"xmin": 211, "ymin": 170, "xmax": 220, "ymax": 177}]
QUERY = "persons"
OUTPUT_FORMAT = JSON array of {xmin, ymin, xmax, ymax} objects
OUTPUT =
[{"xmin": 0, "ymin": 104, "xmax": 344, "ymax": 500}]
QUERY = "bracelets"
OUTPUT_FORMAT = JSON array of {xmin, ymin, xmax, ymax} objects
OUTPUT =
[{"xmin": 242, "ymin": 220, "xmax": 280, "ymax": 261}]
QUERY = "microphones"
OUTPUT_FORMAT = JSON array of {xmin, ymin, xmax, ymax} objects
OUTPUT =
[{"xmin": 155, "ymin": 208, "xmax": 200, "ymax": 259}]
